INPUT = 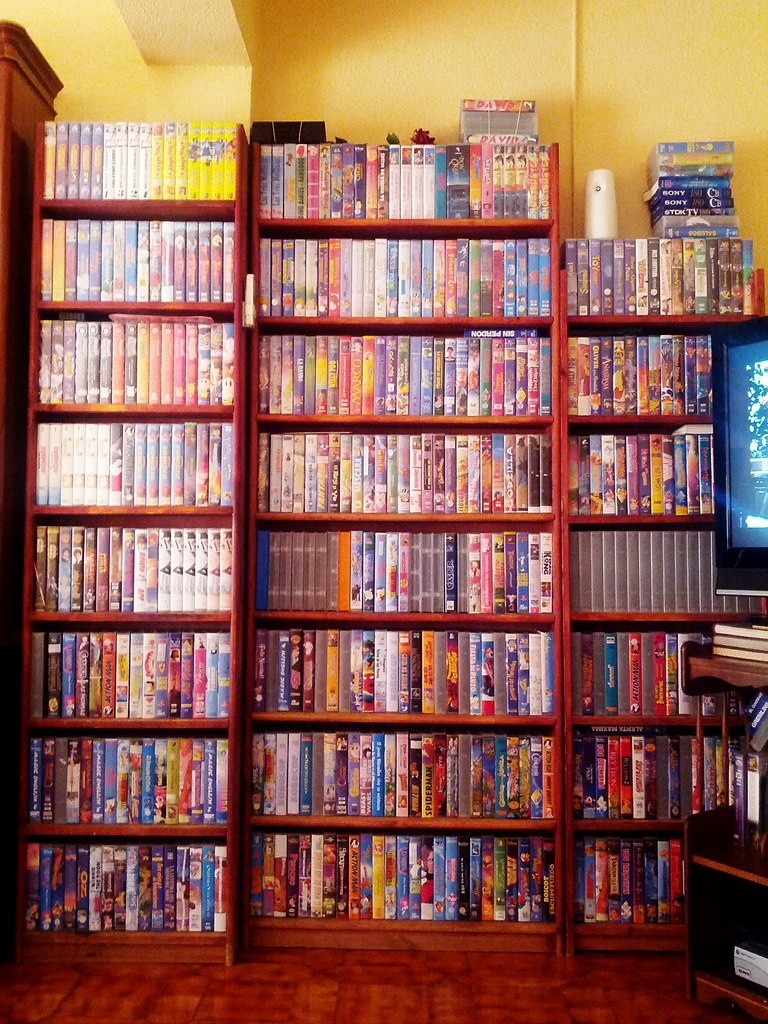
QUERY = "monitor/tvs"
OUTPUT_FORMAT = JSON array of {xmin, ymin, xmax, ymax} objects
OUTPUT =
[{"xmin": 711, "ymin": 314, "xmax": 768, "ymax": 598}]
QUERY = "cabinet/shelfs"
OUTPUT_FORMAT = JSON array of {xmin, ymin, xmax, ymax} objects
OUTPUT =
[
  {"xmin": 237, "ymin": 141, "xmax": 563, "ymax": 958},
  {"xmin": 662, "ymin": 639, "xmax": 768, "ymax": 1024},
  {"xmin": 559, "ymin": 239, "xmax": 759, "ymax": 958},
  {"xmin": 11, "ymin": 121, "xmax": 233, "ymax": 971}
]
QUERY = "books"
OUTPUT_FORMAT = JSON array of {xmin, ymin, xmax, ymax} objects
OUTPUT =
[{"xmin": 37, "ymin": 122, "xmax": 767, "ymax": 936}]
